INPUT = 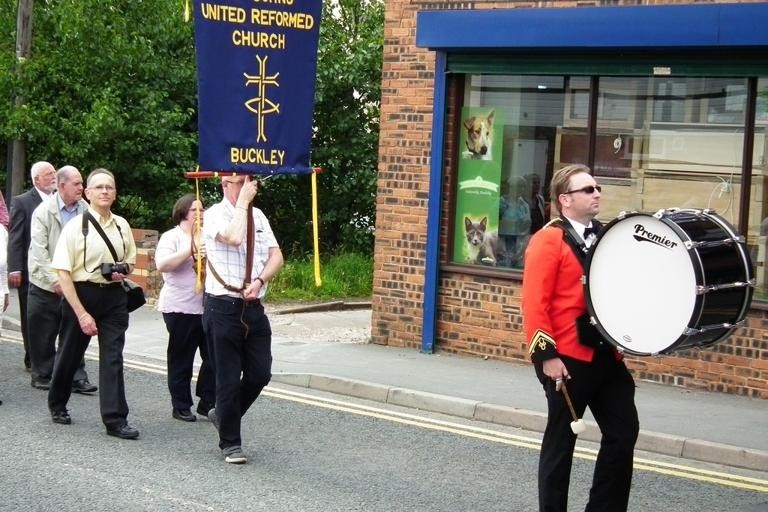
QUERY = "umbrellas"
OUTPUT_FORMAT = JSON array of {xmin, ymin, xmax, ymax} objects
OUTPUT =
[
  {"xmin": 107, "ymin": 424, "xmax": 139, "ymax": 438},
  {"xmin": 30, "ymin": 380, "xmax": 50, "ymax": 389},
  {"xmin": 49, "ymin": 406, "xmax": 70, "ymax": 423},
  {"xmin": 72, "ymin": 380, "xmax": 97, "ymax": 392}
]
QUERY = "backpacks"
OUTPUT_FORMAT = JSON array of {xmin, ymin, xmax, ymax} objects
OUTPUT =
[{"xmin": 579, "ymin": 208, "xmax": 755, "ymax": 357}]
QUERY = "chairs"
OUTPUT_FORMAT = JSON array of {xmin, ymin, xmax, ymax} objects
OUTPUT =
[
  {"xmin": 196, "ymin": 399, "xmax": 221, "ymax": 434},
  {"xmin": 222, "ymin": 444, "xmax": 248, "ymax": 463},
  {"xmin": 172, "ymin": 409, "xmax": 195, "ymax": 422}
]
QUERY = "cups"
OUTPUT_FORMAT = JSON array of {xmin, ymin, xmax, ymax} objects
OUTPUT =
[{"xmin": 564, "ymin": 186, "xmax": 601, "ymax": 194}]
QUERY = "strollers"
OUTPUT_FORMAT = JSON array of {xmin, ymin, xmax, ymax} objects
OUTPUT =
[{"xmin": 584, "ymin": 229, "xmax": 597, "ymax": 239}]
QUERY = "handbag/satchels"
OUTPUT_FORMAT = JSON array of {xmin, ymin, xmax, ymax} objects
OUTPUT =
[
  {"xmin": 79, "ymin": 312, "xmax": 88, "ymax": 321},
  {"xmin": 236, "ymin": 207, "xmax": 249, "ymax": 210},
  {"xmin": 254, "ymin": 277, "xmax": 264, "ymax": 285}
]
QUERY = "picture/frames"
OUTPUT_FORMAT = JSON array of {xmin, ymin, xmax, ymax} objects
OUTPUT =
[{"xmin": 498, "ymin": 220, "xmax": 533, "ymax": 268}]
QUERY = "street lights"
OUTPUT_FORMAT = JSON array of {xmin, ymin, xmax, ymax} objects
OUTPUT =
[{"xmin": 121, "ymin": 278, "xmax": 146, "ymax": 312}]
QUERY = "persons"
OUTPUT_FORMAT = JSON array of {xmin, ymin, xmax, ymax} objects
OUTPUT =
[
  {"xmin": 153, "ymin": 193, "xmax": 216, "ymax": 422},
  {"xmin": 0, "ymin": 223, "xmax": 11, "ymax": 405},
  {"xmin": 26, "ymin": 165, "xmax": 97, "ymax": 392},
  {"xmin": 6, "ymin": 160, "xmax": 58, "ymax": 372},
  {"xmin": 521, "ymin": 163, "xmax": 639, "ymax": 512},
  {"xmin": 495, "ymin": 173, "xmax": 546, "ymax": 266},
  {"xmin": 1, "ymin": 191, "xmax": 8, "ymax": 225},
  {"xmin": 48, "ymin": 168, "xmax": 139, "ymax": 438},
  {"xmin": 202, "ymin": 175, "xmax": 283, "ymax": 463}
]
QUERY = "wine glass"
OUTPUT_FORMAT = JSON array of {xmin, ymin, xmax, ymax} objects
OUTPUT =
[
  {"xmin": 556, "ymin": 379, "xmax": 562, "ymax": 382},
  {"xmin": 565, "ymin": 373, "xmax": 569, "ymax": 378}
]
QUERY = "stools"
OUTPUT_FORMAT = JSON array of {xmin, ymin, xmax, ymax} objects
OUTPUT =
[
  {"xmin": 207, "ymin": 292, "xmax": 259, "ymax": 307},
  {"xmin": 98, "ymin": 283, "xmax": 112, "ymax": 288}
]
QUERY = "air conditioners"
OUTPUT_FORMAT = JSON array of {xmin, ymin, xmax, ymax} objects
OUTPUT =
[
  {"xmin": 462, "ymin": 109, "xmax": 495, "ymax": 160},
  {"xmin": 465, "ymin": 216, "xmax": 498, "ymax": 267}
]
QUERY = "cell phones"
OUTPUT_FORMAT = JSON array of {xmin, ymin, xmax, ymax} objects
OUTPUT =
[{"xmin": 101, "ymin": 263, "xmax": 130, "ymax": 281}]
